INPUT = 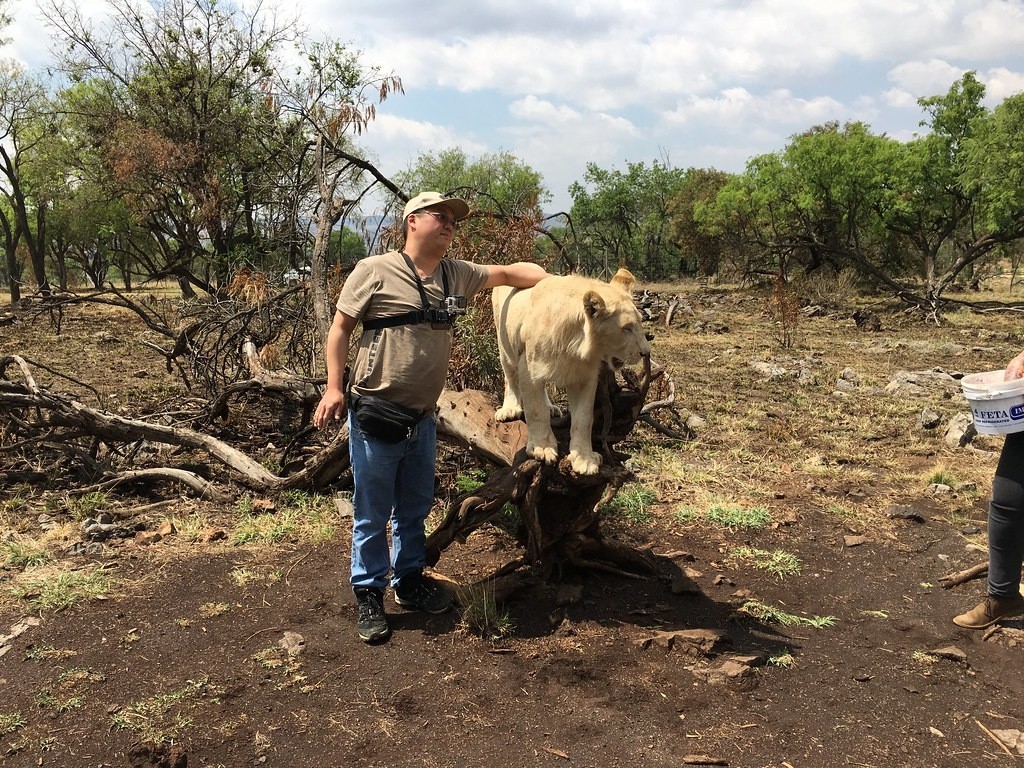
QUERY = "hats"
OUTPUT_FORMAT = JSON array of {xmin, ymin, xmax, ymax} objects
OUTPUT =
[{"xmin": 403, "ymin": 191, "xmax": 470, "ymax": 219}]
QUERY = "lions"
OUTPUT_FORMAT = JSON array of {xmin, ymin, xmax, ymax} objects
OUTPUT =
[{"xmin": 492, "ymin": 268, "xmax": 650, "ymax": 475}]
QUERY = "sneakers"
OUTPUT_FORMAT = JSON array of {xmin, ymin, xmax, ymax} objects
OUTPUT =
[
  {"xmin": 953, "ymin": 593, "xmax": 1024, "ymax": 629},
  {"xmin": 395, "ymin": 575, "xmax": 454, "ymax": 613},
  {"xmin": 356, "ymin": 588, "xmax": 390, "ymax": 641}
]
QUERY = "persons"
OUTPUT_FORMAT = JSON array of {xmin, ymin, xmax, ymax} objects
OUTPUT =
[
  {"xmin": 313, "ymin": 191, "xmax": 554, "ymax": 643},
  {"xmin": 953, "ymin": 350, "xmax": 1024, "ymax": 628}
]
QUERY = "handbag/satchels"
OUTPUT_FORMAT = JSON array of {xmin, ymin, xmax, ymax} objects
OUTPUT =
[{"xmin": 350, "ymin": 394, "xmax": 435, "ymax": 444}]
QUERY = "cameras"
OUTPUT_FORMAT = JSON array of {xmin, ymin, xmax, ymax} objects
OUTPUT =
[{"xmin": 444, "ymin": 294, "xmax": 467, "ymax": 317}]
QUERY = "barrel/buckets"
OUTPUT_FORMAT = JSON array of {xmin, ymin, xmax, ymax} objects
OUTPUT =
[{"xmin": 961, "ymin": 370, "xmax": 1024, "ymax": 434}]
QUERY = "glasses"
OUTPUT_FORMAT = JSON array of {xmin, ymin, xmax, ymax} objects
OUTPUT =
[{"xmin": 420, "ymin": 209, "xmax": 460, "ymax": 230}]
